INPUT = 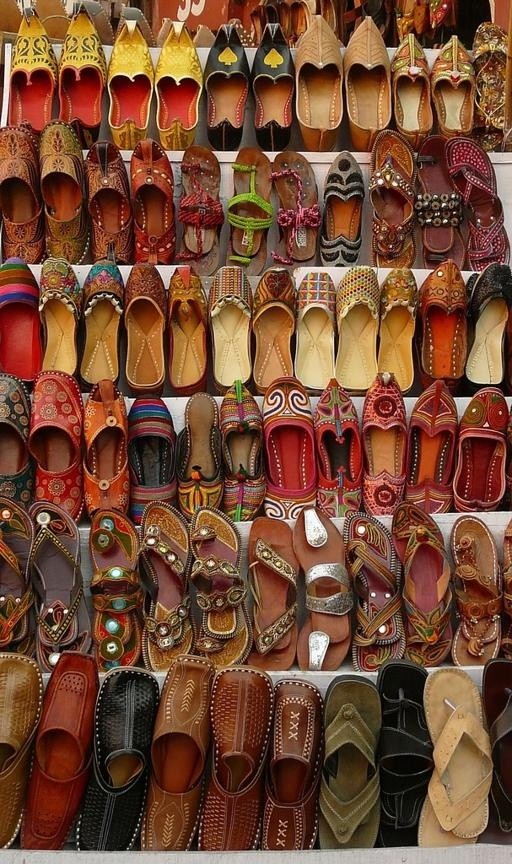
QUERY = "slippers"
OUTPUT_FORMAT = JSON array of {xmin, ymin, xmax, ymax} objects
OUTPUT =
[
  {"xmin": 1, "ymin": 499, "xmax": 512, "ymax": 672},
  {"xmin": 0, "ymin": 369, "xmax": 511, "ymax": 518},
  {"xmin": 1, "ymin": 254, "xmax": 418, "ymax": 400},
  {"xmin": 1, "ymin": 651, "xmax": 326, "ymax": 850},
  {"xmin": 471, "ymin": 20, "xmax": 512, "ymax": 154},
  {"xmin": 179, "ymin": 143, "xmax": 319, "ymax": 276}
]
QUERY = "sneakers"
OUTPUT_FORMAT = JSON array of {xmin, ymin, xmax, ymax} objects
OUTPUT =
[
  {"xmin": 323, "ymin": 147, "xmax": 368, "ymax": 269},
  {"xmin": 2, "ymin": 117, "xmax": 176, "ymax": 266}
]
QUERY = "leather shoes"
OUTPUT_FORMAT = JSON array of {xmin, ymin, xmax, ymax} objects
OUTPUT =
[
  {"xmin": 5, "ymin": 0, "xmax": 477, "ymax": 159},
  {"xmin": 420, "ymin": 256, "xmax": 512, "ymax": 392}
]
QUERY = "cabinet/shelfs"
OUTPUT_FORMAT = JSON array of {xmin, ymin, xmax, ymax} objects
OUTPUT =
[{"xmin": 1, "ymin": 0, "xmax": 510, "ymax": 864}]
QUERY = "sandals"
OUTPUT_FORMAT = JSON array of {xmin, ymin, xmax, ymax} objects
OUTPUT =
[
  {"xmin": 315, "ymin": 657, "xmax": 512, "ymax": 849},
  {"xmin": 368, "ymin": 121, "xmax": 508, "ymax": 275}
]
